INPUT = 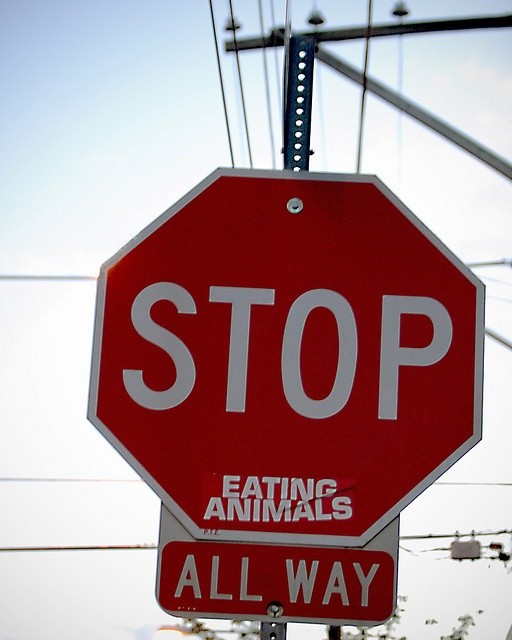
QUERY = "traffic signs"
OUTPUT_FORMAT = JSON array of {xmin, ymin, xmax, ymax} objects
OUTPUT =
[{"xmin": 158, "ymin": 540, "xmax": 395, "ymax": 626}]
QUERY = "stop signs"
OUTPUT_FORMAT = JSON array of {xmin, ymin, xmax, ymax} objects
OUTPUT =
[{"xmin": 86, "ymin": 166, "xmax": 488, "ymax": 548}]
